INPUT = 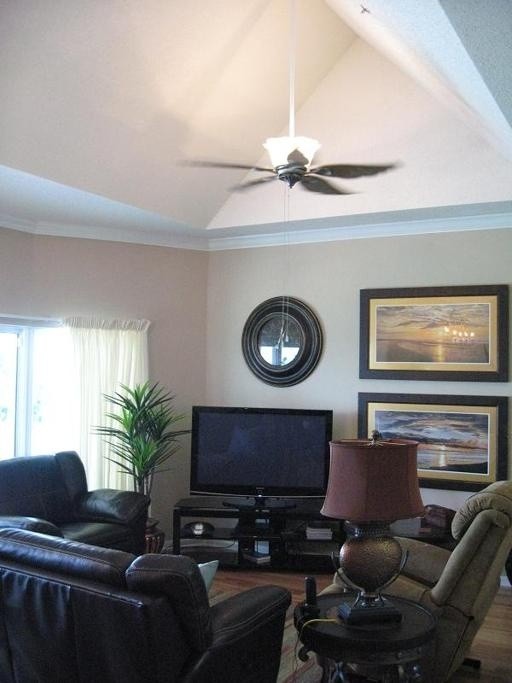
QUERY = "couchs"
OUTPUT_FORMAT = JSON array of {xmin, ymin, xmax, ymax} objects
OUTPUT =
[{"xmin": 0, "ymin": 513, "xmax": 294, "ymax": 682}]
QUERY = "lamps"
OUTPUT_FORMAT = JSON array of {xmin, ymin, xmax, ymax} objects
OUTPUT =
[
  {"xmin": 261, "ymin": 134, "xmax": 320, "ymax": 170},
  {"xmin": 319, "ymin": 434, "xmax": 427, "ymax": 634}
]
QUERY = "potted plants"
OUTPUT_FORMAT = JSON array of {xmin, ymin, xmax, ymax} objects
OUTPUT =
[{"xmin": 92, "ymin": 379, "xmax": 195, "ymax": 555}]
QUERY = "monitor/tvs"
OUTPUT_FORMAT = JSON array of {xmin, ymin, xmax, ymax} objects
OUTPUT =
[{"xmin": 189, "ymin": 404, "xmax": 334, "ymax": 509}]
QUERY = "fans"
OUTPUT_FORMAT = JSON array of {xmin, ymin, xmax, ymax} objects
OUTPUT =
[{"xmin": 178, "ymin": 1, "xmax": 392, "ymax": 196}]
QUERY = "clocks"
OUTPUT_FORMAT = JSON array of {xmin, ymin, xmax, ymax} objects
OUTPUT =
[{"xmin": 241, "ymin": 295, "xmax": 324, "ymax": 388}]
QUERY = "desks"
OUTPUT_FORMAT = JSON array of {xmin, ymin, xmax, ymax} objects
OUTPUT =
[
  {"xmin": 293, "ymin": 592, "xmax": 438, "ymax": 683},
  {"xmin": 388, "ymin": 514, "xmax": 456, "ymax": 549}
]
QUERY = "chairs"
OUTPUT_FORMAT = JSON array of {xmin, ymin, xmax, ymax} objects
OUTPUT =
[
  {"xmin": 313, "ymin": 476, "xmax": 511, "ymax": 683},
  {"xmin": 1, "ymin": 450, "xmax": 153, "ymax": 556}
]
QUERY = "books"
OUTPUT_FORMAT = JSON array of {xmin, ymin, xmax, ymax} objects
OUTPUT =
[
  {"xmin": 307, "ymin": 526, "xmax": 332, "ymax": 531},
  {"xmin": 244, "ymin": 549, "xmax": 272, "ymax": 560},
  {"xmin": 305, "ymin": 529, "xmax": 333, "ymax": 534},
  {"xmin": 306, "ymin": 536, "xmax": 333, "ymax": 539},
  {"xmin": 306, "ymin": 533, "xmax": 333, "ymax": 536}
]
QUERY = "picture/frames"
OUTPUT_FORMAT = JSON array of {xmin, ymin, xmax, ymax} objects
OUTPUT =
[
  {"xmin": 356, "ymin": 392, "xmax": 510, "ymax": 492},
  {"xmin": 358, "ymin": 283, "xmax": 508, "ymax": 383}
]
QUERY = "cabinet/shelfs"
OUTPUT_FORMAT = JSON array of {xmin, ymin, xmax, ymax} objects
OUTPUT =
[{"xmin": 170, "ymin": 497, "xmax": 346, "ymax": 575}]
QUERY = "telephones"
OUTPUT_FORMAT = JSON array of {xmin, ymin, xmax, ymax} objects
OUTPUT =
[{"xmin": 303, "ymin": 576, "xmax": 320, "ymax": 614}]
